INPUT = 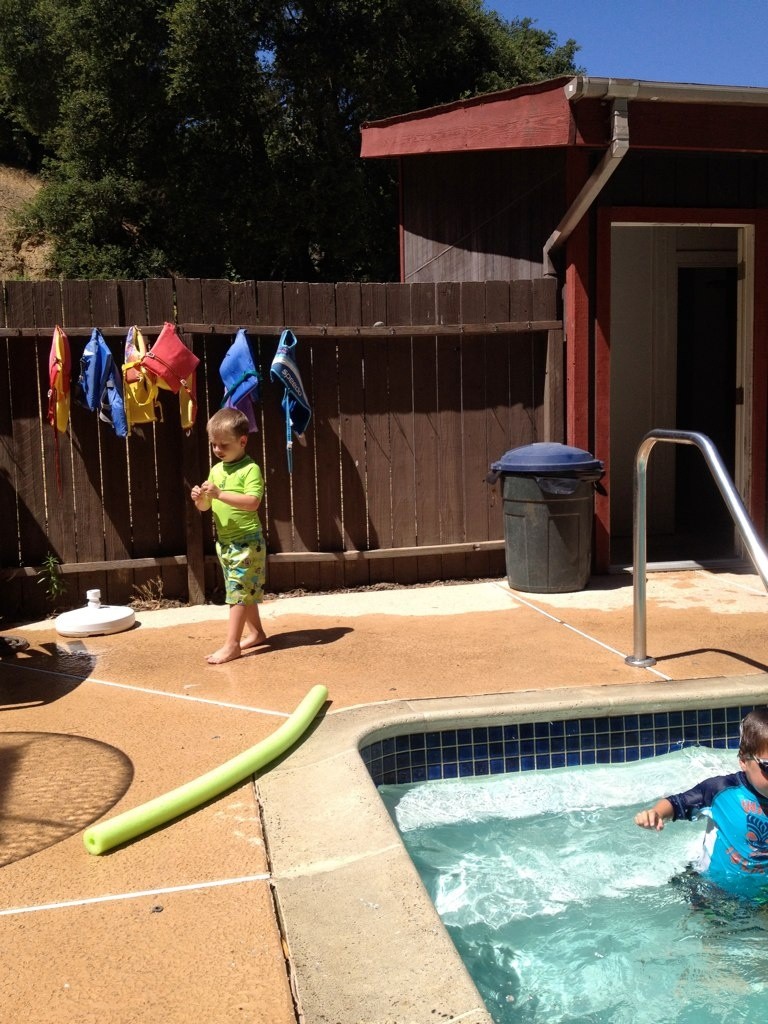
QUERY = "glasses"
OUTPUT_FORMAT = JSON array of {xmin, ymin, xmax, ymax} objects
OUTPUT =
[{"xmin": 751, "ymin": 752, "xmax": 768, "ymax": 774}]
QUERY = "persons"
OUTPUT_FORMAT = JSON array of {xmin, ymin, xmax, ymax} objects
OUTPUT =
[
  {"xmin": 633, "ymin": 707, "xmax": 767, "ymax": 927},
  {"xmin": 192, "ymin": 407, "xmax": 274, "ymax": 665}
]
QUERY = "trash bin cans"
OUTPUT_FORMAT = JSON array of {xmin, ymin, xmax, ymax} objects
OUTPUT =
[{"xmin": 484, "ymin": 442, "xmax": 608, "ymax": 593}]
